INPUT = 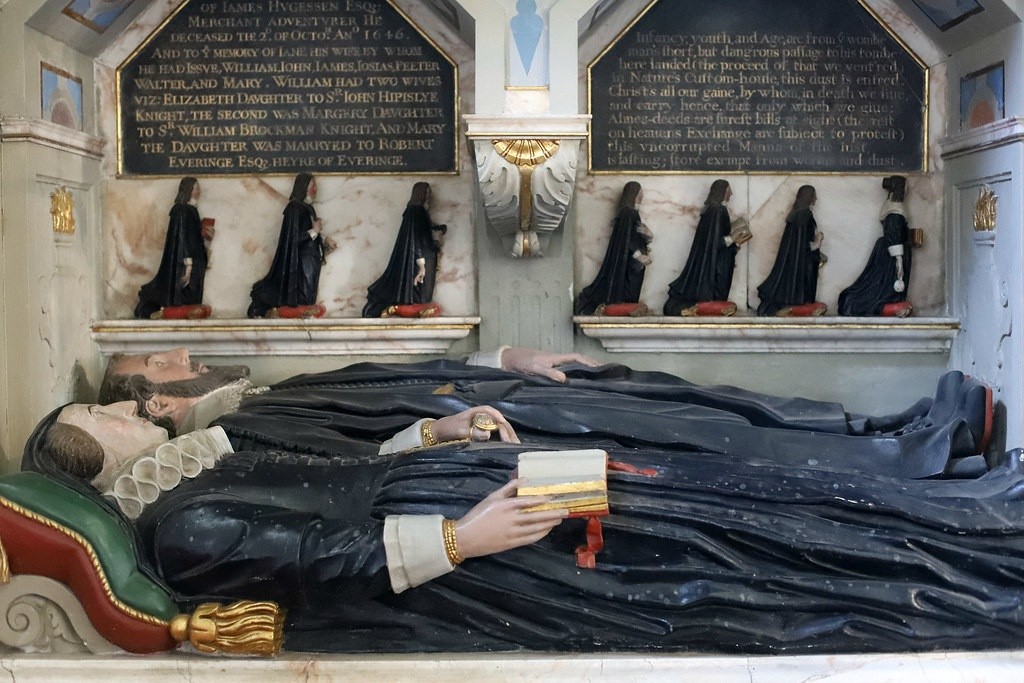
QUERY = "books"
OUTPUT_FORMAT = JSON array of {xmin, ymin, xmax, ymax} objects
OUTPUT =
[
  {"xmin": 201, "ymin": 217, "xmax": 215, "ymax": 238},
  {"xmin": 517, "ymin": 448, "xmax": 609, "ymax": 519},
  {"xmin": 731, "ymin": 217, "xmax": 753, "ymax": 245}
]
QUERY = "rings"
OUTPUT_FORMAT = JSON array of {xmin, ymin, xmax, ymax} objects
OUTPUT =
[{"xmin": 470, "ymin": 412, "xmax": 499, "ymax": 439}]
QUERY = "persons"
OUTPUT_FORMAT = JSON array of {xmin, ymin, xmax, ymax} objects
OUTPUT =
[
  {"xmin": 664, "ymin": 180, "xmax": 745, "ymax": 316},
  {"xmin": 837, "ymin": 175, "xmax": 912, "ymax": 316},
  {"xmin": 576, "ymin": 180, "xmax": 654, "ymax": 317},
  {"xmin": 95, "ymin": 343, "xmax": 996, "ymax": 482},
  {"xmin": 756, "ymin": 185, "xmax": 823, "ymax": 317},
  {"xmin": 362, "ymin": 182, "xmax": 448, "ymax": 318},
  {"xmin": 248, "ymin": 174, "xmax": 337, "ymax": 319},
  {"xmin": 22, "ymin": 396, "xmax": 1024, "ymax": 655},
  {"xmin": 135, "ymin": 177, "xmax": 215, "ymax": 319}
]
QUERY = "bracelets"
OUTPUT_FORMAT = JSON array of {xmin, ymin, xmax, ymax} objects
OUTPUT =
[
  {"xmin": 443, "ymin": 518, "xmax": 462, "ymax": 566},
  {"xmin": 421, "ymin": 420, "xmax": 436, "ymax": 447}
]
